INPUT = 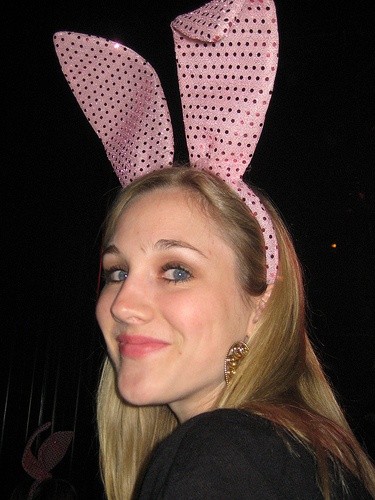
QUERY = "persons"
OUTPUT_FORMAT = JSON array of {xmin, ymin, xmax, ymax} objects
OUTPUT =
[
  {"xmin": 10, "ymin": 422, "xmax": 80, "ymax": 500},
  {"xmin": 53, "ymin": 1, "xmax": 375, "ymax": 500}
]
